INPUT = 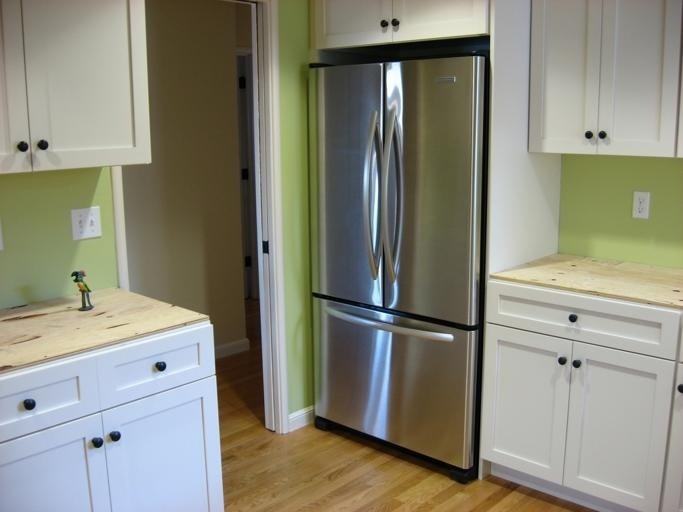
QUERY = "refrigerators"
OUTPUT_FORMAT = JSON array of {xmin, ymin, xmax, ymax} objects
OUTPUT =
[{"xmin": 309, "ymin": 36, "xmax": 488, "ymax": 484}]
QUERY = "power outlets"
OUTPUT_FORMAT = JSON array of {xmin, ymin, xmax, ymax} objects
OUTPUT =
[
  {"xmin": 629, "ymin": 190, "xmax": 651, "ymax": 220},
  {"xmin": 69, "ymin": 206, "xmax": 104, "ymax": 242}
]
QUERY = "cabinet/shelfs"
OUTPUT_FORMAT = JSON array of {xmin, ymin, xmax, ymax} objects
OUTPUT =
[
  {"xmin": 527, "ymin": 1, "xmax": 682, "ymax": 158},
  {"xmin": 1, "ymin": 325, "xmax": 224, "ymax": 512},
  {"xmin": 0, "ymin": 1, "xmax": 152, "ymax": 174},
  {"xmin": 478, "ymin": 277, "xmax": 682, "ymax": 511},
  {"xmin": 310, "ymin": 1, "xmax": 491, "ymax": 51}
]
